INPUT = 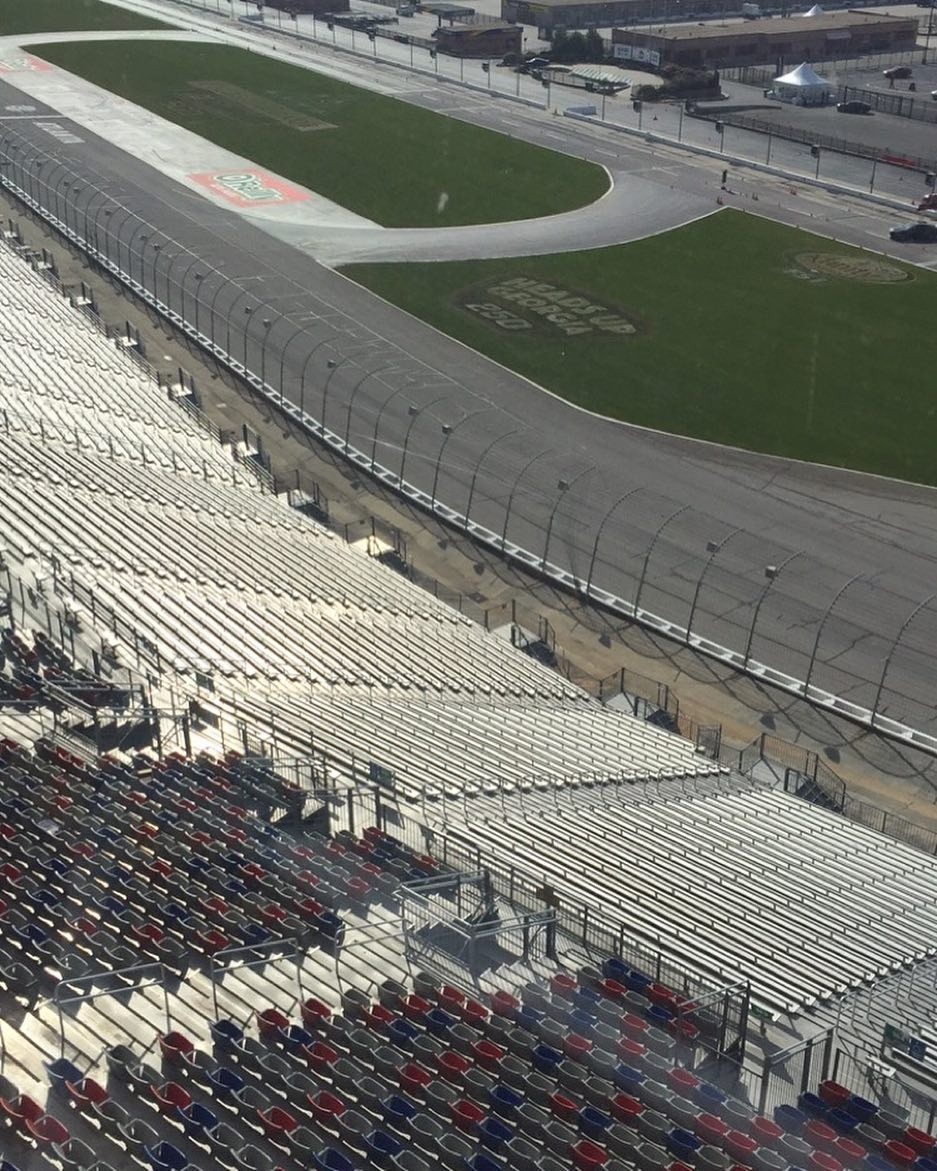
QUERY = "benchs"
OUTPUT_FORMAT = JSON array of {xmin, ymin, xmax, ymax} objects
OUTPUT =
[{"xmin": 1, "ymin": 239, "xmax": 936, "ymax": 1016}]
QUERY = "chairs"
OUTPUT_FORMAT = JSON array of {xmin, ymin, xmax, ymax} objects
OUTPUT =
[{"xmin": 1, "ymin": 628, "xmax": 936, "ymax": 1171}]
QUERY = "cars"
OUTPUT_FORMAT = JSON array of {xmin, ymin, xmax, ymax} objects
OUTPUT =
[
  {"xmin": 526, "ymin": 57, "xmax": 549, "ymax": 66},
  {"xmin": 890, "ymin": 221, "xmax": 937, "ymax": 244},
  {"xmin": 396, "ymin": 4, "xmax": 414, "ymax": 17},
  {"xmin": 883, "ymin": 66, "xmax": 912, "ymax": 78},
  {"xmin": 837, "ymin": 100, "xmax": 871, "ymax": 114}
]
